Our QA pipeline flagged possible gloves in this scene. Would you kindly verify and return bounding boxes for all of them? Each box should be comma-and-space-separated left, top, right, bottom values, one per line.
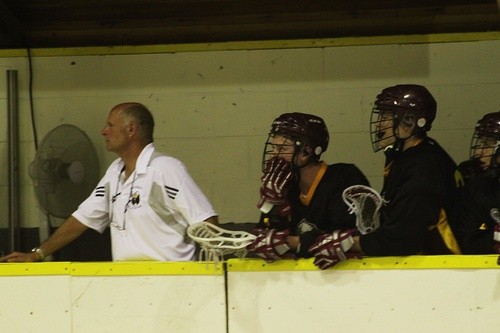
257, 156, 303, 224
246, 228, 298, 264
308, 227, 363, 271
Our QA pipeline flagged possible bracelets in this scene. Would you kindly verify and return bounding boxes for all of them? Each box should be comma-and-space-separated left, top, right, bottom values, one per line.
32, 248, 44, 260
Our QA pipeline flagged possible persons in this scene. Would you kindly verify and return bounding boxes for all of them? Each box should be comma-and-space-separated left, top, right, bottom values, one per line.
311, 84, 477, 270
246, 113, 371, 262
457, 111, 500, 254
0, 103, 219, 262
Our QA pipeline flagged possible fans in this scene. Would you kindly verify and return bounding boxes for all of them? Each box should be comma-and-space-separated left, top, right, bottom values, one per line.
30, 125, 100, 262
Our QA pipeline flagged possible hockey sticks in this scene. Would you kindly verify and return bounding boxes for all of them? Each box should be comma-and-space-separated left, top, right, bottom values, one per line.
341, 184, 385, 235
185, 221, 259, 257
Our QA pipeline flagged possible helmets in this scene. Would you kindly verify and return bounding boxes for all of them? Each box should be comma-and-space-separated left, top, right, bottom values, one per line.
369, 84, 437, 153
262, 112, 329, 173
470, 111, 500, 177
